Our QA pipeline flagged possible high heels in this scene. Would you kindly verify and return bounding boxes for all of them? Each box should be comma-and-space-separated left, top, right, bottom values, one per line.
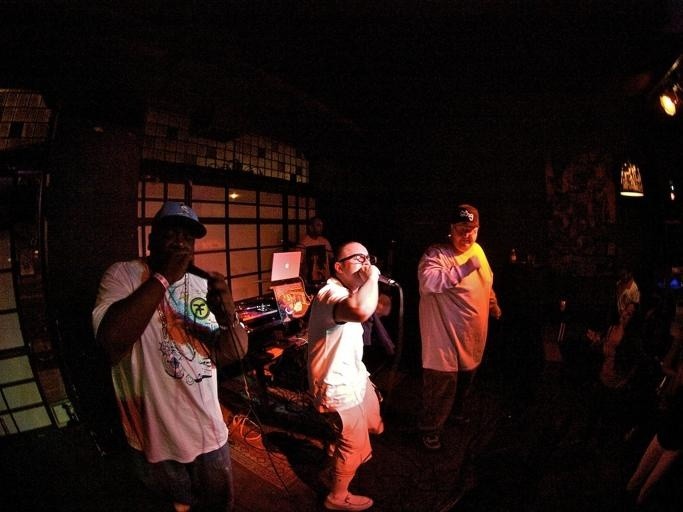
328, 443, 372, 464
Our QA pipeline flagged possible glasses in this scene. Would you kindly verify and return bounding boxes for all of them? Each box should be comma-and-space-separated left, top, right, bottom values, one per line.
339, 254, 377, 265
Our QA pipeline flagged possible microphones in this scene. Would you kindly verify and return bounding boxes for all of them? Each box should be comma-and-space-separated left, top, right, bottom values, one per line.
379, 275, 397, 287
188, 264, 211, 280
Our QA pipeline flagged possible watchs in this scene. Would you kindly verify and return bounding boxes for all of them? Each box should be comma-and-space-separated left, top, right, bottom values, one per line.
219, 312, 240, 331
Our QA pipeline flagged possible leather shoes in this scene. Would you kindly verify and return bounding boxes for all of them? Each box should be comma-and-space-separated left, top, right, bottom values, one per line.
324, 491, 373, 511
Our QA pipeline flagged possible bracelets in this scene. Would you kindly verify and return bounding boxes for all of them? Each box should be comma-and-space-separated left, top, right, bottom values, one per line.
149, 268, 174, 294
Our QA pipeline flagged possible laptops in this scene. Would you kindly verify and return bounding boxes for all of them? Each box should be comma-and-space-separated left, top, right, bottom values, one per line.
252, 252, 301, 282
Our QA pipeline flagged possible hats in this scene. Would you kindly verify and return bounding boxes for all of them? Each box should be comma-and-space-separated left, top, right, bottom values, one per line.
451, 204, 479, 224
151, 202, 206, 239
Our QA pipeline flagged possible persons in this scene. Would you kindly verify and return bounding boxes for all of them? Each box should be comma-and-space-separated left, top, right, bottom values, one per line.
295, 217, 334, 281
306, 240, 392, 512
417, 203, 502, 449
586, 261, 683, 512
85, 199, 249, 512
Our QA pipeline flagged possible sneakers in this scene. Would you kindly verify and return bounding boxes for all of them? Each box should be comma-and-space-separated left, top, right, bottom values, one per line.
453, 415, 470, 422
423, 435, 441, 449
